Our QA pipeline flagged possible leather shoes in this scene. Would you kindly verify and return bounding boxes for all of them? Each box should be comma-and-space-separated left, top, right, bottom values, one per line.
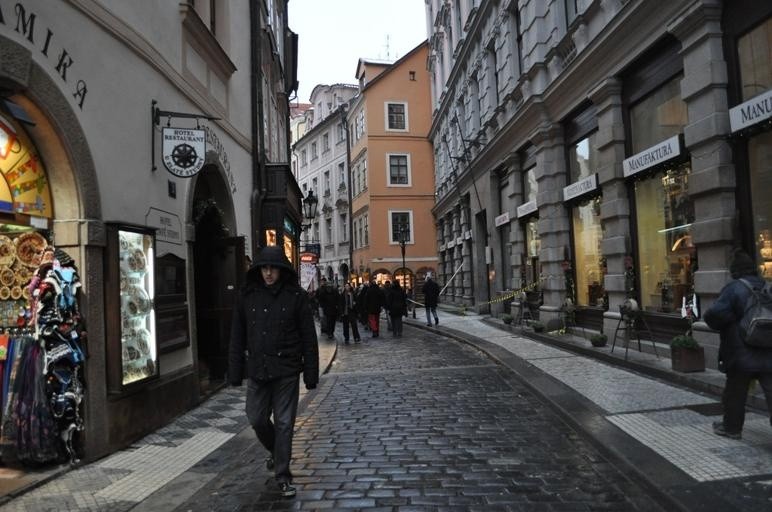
427, 323, 433, 327
327, 333, 334, 339
435, 317, 439, 325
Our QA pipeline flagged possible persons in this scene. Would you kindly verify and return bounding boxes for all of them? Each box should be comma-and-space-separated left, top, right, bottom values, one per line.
422, 277, 440, 327
342, 284, 360, 344
316, 281, 341, 338
702, 255, 772, 438
357, 277, 407, 340
226, 248, 318, 500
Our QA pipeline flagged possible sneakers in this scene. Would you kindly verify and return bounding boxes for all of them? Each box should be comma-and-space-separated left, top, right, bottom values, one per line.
266, 454, 275, 471
712, 421, 741, 440
345, 337, 350, 345
354, 337, 361, 342
278, 481, 297, 497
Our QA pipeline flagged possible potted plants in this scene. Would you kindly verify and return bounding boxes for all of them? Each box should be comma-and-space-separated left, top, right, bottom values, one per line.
591, 334, 608, 346
670, 335, 706, 373
532, 320, 545, 332
502, 315, 513, 324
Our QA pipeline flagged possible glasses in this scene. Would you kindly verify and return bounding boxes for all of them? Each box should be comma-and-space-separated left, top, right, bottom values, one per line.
261, 265, 281, 273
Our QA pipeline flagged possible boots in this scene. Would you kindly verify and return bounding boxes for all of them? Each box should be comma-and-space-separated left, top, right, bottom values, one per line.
372, 331, 378, 338
377, 330, 379, 336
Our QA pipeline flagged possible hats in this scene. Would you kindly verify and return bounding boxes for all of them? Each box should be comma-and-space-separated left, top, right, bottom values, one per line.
326, 284, 332, 286
27, 247, 85, 464
729, 247, 758, 280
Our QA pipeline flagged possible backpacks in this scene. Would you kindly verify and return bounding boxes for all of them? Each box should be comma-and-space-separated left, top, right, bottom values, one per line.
738, 276, 771, 352
